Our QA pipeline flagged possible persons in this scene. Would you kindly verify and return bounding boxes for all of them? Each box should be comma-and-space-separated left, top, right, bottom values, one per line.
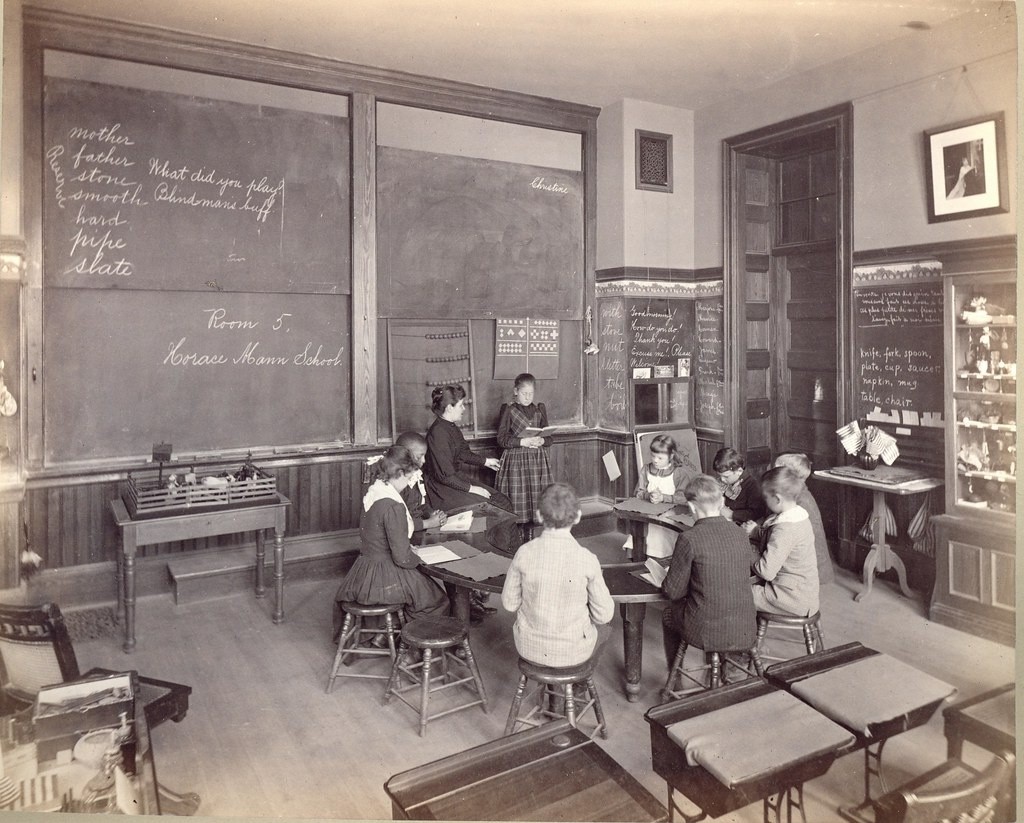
622, 435, 690, 573
660, 475, 757, 700
333, 445, 450, 648
946, 157, 975, 200
495, 373, 554, 538
501, 482, 615, 720
713, 448, 833, 618
397, 431, 498, 626
423, 385, 520, 555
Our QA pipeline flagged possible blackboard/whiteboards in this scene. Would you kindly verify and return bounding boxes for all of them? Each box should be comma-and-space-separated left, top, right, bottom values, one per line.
849, 274, 948, 430
595, 281, 724, 434
15, 0, 603, 475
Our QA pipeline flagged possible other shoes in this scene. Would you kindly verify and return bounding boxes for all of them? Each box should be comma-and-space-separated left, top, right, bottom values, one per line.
371, 634, 389, 649
549, 700, 565, 722
659, 674, 683, 696
402, 649, 422, 683
574, 697, 587, 716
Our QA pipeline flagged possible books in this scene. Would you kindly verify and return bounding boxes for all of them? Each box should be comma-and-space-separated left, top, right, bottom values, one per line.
518, 426, 558, 436
440, 510, 474, 531
641, 558, 670, 588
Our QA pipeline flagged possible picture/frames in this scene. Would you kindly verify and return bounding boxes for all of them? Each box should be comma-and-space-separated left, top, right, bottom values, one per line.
922, 110, 1010, 224
634, 128, 673, 192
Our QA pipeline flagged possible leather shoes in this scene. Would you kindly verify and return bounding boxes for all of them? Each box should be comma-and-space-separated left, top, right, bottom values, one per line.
452, 597, 498, 619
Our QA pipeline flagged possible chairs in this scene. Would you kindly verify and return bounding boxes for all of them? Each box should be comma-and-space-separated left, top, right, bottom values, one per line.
872, 745, 1017, 823
0, 601, 192, 729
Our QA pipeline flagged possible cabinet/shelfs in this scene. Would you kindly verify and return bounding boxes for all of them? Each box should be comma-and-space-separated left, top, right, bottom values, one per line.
930, 269, 1016, 648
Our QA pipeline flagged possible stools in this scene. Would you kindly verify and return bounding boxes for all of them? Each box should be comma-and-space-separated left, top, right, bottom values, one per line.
747, 610, 823, 679
502, 656, 608, 741
660, 637, 763, 704
380, 615, 490, 734
325, 600, 407, 696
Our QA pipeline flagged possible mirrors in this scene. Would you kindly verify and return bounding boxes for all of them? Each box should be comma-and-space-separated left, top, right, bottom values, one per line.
628, 378, 695, 430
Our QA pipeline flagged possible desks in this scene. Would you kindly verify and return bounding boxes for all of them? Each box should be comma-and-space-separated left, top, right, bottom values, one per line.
943, 681, 1016, 759
382, 717, 668, 822
764, 640, 959, 822
644, 675, 857, 822
812, 463, 945, 602
410, 495, 769, 701
109, 491, 292, 653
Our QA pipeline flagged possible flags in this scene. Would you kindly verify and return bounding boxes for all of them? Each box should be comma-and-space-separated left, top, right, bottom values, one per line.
837, 419, 900, 466
